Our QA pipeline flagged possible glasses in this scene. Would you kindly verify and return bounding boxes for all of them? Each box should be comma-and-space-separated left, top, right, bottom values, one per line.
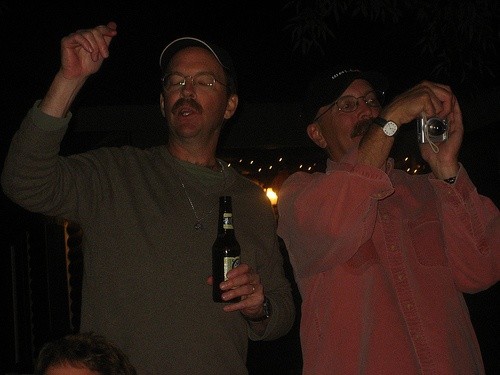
164, 72, 226, 93
313, 91, 386, 124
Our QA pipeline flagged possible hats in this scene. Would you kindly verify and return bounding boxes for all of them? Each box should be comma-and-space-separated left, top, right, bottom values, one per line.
305, 60, 375, 126
159, 36, 237, 89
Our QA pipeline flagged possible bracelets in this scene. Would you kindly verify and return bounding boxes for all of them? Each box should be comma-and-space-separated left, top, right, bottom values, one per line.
444, 176, 457, 184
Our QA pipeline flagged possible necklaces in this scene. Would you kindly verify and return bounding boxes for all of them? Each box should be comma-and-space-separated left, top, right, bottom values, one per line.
175, 175, 219, 232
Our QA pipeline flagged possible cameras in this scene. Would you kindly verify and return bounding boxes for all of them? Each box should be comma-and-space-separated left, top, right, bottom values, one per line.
416, 112, 449, 142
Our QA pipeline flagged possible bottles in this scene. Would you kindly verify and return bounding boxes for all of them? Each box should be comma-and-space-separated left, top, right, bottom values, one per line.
212, 196, 240, 304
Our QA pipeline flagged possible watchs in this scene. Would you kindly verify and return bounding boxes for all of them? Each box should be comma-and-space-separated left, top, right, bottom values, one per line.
372, 117, 399, 137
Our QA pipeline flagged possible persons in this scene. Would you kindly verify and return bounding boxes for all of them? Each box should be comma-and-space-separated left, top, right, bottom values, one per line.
1, 19, 296, 375
33, 328, 137, 375
275, 65, 500, 374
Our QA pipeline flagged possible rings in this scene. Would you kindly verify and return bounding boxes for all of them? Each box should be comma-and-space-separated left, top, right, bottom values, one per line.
251, 285, 256, 293
